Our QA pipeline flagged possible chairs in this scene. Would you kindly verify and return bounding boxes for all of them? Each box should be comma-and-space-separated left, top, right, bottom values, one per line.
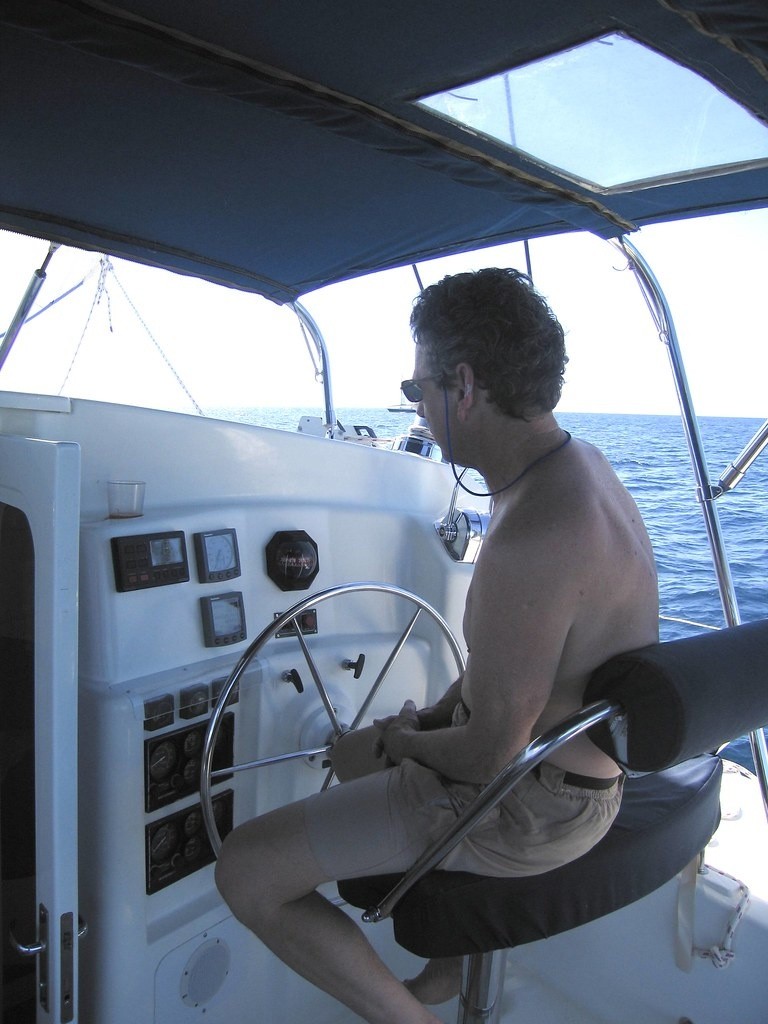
340, 617, 768, 1024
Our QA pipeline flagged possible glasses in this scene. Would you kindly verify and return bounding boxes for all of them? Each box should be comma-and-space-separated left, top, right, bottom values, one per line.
400, 373, 456, 403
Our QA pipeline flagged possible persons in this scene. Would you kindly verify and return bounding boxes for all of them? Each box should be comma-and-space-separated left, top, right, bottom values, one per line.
215, 267, 657, 1024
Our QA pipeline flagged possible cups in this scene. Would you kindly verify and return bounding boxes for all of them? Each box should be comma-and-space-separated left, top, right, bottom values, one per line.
106, 480, 146, 518
